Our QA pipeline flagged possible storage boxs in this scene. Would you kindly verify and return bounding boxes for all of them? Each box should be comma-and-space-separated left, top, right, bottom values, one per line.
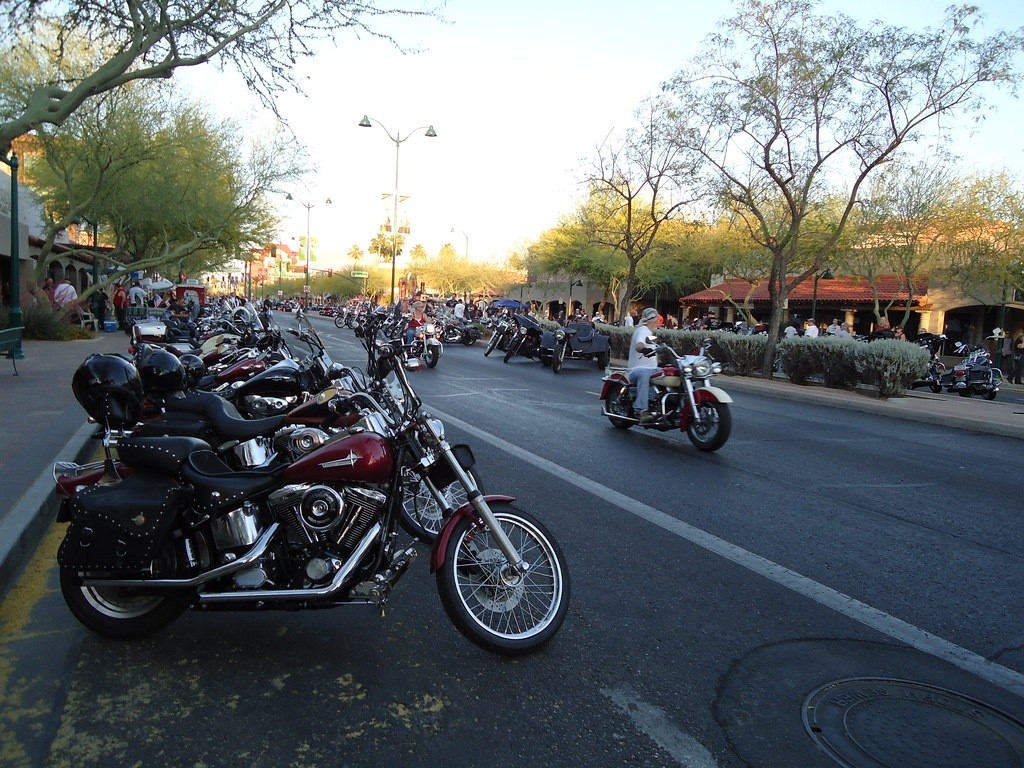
141, 418, 207, 440
58, 482, 188, 574
103, 321, 117, 332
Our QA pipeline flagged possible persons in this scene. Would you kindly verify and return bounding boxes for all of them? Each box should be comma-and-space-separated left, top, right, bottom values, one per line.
111, 283, 128, 330
874, 316, 891, 331
95, 288, 108, 330
825, 319, 852, 339
895, 325, 906, 342
803, 318, 819, 338
454, 299, 465, 326
784, 321, 798, 338
626, 308, 685, 424
485, 302, 496, 317
1006, 333, 1024, 384
263, 294, 273, 313
682, 310, 734, 331
624, 311, 679, 330
129, 281, 147, 306
165, 297, 190, 324
42, 277, 78, 312
551, 308, 605, 327
466, 298, 478, 322
445, 293, 458, 314
998, 329, 1014, 376
394, 291, 435, 345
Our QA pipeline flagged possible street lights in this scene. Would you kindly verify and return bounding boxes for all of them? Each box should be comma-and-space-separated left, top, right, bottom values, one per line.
519, 280, 534, 313
359, 114, 437, 309
449, 229, 471, 273
568, 277, 584, 318
654, 276, 672, 310
278, 234, 295, 290
286, 191, 333, 314
809, 266, 836, 320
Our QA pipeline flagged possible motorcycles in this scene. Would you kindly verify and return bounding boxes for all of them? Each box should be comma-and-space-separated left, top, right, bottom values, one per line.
48, 274, 1005, 657
598, 334, 733, 452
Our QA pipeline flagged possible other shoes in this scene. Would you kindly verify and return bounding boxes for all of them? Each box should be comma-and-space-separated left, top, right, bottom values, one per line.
640, 411, 653, 423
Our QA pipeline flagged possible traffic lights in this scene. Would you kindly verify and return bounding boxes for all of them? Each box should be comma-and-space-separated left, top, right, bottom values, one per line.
327, 269, 333, 278
304, 265, 308, 274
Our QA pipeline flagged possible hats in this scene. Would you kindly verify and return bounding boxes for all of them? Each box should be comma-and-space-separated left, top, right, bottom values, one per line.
65, 277, 73, 283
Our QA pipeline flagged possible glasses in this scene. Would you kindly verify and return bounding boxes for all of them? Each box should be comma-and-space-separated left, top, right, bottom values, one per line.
418, 305, 423, 308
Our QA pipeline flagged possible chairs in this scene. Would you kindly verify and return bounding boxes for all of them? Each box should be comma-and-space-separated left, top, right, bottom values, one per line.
76, 306, 99, 333
0, 327, 25, 376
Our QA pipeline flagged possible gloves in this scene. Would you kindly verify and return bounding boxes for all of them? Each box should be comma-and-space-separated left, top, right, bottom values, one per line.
639, 348, 655, 358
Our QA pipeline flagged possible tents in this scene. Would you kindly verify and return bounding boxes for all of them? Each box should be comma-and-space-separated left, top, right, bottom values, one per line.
486, 299, 530, 309
